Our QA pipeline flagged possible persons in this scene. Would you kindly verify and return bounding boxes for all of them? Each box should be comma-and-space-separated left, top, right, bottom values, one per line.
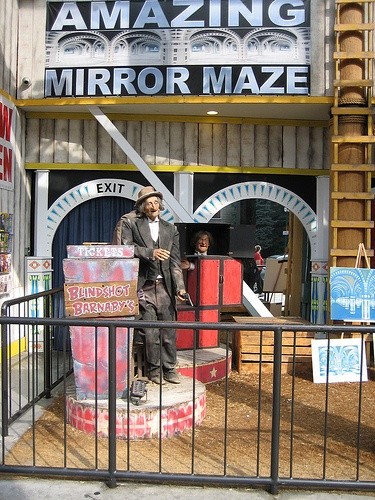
253, 244, 264, 293
180, 230, 222, 256
121, 187, 187, 384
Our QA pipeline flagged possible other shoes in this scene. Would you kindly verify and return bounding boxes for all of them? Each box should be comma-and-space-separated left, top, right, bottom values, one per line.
150, 376, 166, 385
164, 372, 181, 384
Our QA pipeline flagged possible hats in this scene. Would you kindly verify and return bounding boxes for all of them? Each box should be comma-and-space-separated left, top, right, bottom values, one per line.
135, 186, 163, 206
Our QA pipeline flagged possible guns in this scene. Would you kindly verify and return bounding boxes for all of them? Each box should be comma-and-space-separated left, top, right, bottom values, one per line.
181, 293, 193, 306
